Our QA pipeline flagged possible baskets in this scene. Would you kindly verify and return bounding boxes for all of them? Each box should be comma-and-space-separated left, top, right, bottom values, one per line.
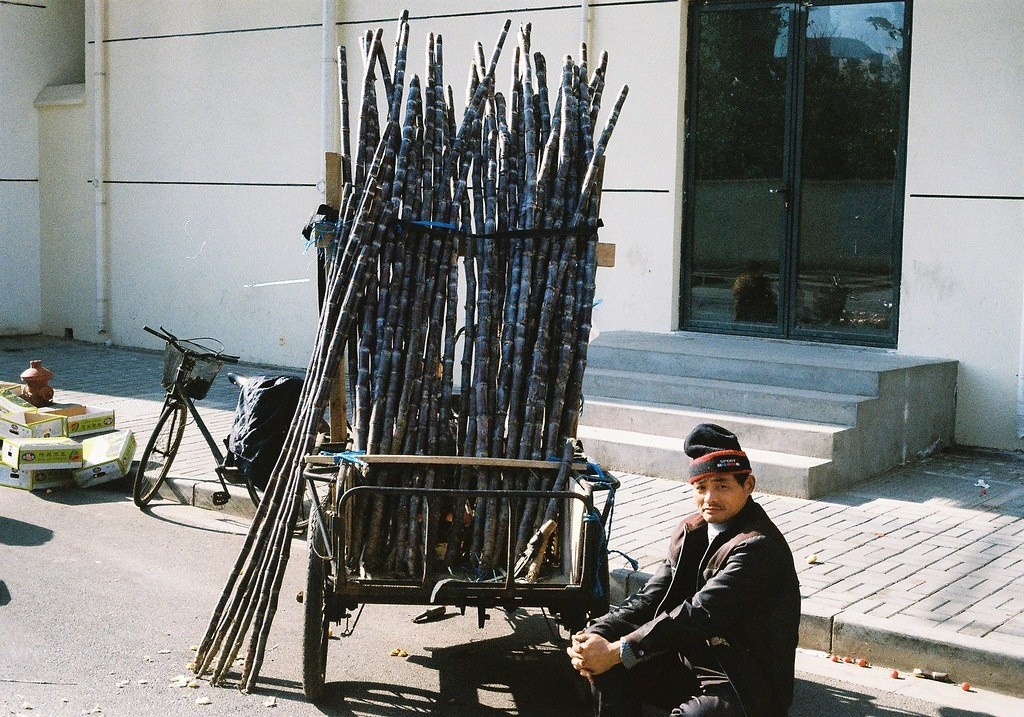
161, 341, 225, 400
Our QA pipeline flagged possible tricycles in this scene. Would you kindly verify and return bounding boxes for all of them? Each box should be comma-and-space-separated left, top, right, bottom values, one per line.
295, 299, 621, 701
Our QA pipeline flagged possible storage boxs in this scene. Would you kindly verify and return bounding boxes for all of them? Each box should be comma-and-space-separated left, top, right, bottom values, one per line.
0, 381, 137, 490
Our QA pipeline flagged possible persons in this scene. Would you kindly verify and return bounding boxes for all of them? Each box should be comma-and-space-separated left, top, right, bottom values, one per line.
565, 422, 801, 717
733, 260, 778, 324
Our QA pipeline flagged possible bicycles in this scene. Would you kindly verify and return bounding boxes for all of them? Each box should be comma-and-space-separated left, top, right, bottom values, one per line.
134, 325, 346, 532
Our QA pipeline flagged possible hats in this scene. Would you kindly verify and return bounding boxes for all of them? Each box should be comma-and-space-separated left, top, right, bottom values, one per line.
684, 423, 752, 485
